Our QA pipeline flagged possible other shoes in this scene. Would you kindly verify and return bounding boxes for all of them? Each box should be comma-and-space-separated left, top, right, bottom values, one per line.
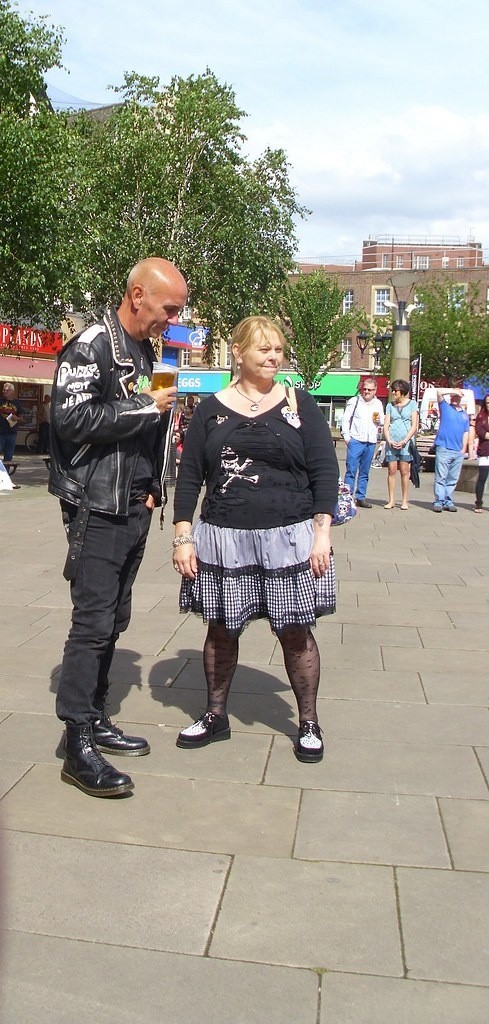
433, 503, 443, 511
384, 501, 395, 509
296, 719, 324, 762
475, 503, 482, 513
357, 499, 372, 508
176, 710, 231, 749
444, 504, 456, 512
400, 500, 408, 510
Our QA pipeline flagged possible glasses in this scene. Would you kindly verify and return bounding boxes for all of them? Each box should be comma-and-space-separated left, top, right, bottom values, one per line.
363, 388, 375, 392
392, 388, 405, 392
2, 389, 13, 392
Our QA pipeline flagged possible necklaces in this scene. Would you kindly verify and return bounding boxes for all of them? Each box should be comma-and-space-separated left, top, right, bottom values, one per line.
235, 381, 273, 411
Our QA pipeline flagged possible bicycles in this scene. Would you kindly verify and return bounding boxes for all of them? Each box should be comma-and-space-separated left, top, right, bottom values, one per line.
25, 430, 40, 452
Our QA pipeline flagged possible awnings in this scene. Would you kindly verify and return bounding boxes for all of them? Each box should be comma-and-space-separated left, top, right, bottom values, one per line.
0, 354, 56, 385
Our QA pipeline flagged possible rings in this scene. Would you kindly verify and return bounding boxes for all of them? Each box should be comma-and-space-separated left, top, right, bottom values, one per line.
319, 561, 323, 565
175, 565, 178, 570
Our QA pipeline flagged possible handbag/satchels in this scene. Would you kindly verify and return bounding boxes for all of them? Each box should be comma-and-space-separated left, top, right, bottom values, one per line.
289, 386, 357, 526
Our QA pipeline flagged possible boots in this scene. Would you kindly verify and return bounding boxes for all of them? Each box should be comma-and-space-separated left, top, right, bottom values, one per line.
96, 694, 150, 756
61, 719, 135, 796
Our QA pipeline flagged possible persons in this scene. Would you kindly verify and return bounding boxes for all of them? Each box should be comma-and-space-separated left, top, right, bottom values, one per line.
0, 382, 24, 472
466, 394, 489, 513
37, 394, 51, 454
48, 257, 188, 798
172, 316, 341, 762
341, 378, 385, 507
432, 388, 470, 512
174, 396, 196, 477
383, 378, 419, 510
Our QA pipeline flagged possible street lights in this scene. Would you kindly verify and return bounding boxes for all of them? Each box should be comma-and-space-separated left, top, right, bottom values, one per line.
355, 328, 393, 379
382, 273, 421, 414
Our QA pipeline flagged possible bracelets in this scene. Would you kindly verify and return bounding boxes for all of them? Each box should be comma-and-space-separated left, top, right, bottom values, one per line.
173, 536, 193, 547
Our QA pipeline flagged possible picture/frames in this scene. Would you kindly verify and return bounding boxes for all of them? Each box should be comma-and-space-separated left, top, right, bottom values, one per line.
16, 400, 39, 431
17, 382, 41, 401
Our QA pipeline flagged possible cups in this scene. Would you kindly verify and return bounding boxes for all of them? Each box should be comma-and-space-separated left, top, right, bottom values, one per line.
373, 412, 379, 422
150, 361, 179, 394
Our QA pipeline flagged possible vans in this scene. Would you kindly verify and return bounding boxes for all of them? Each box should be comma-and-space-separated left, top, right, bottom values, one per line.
418, 386, 476, 433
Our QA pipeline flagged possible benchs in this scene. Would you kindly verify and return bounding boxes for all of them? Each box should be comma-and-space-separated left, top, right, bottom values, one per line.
413, 438, 438, 460
328, 428, 342, 447
